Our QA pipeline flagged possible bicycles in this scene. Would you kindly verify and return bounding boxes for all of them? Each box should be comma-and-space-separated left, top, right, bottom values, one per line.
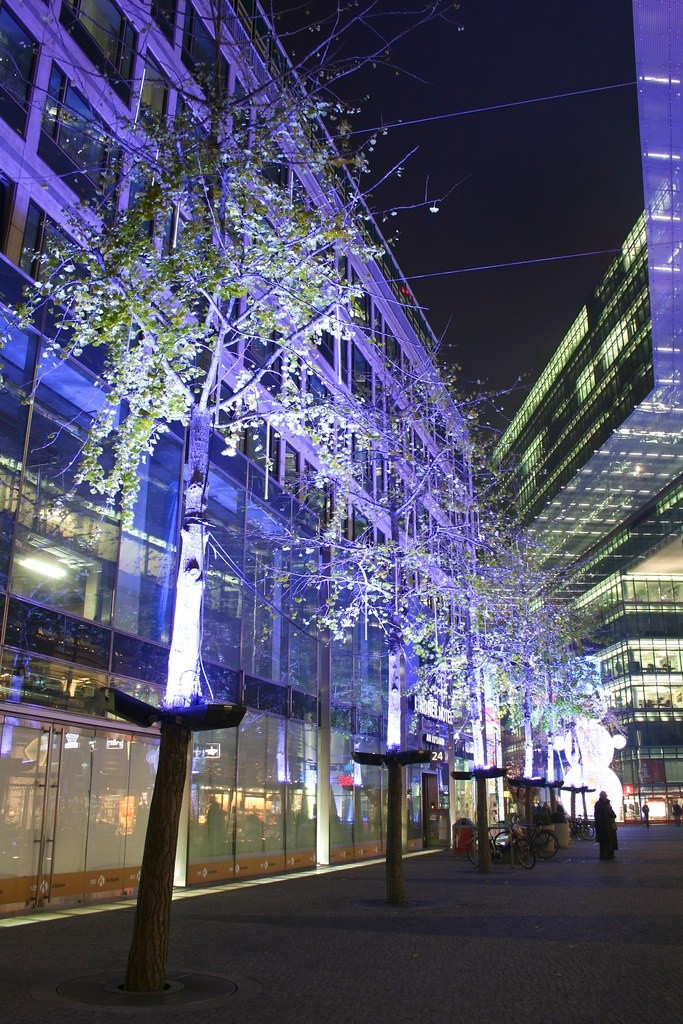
467, 812, 559, 869
568, 815, 596, 841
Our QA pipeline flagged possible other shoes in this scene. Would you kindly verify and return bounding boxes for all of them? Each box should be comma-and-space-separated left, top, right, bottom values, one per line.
599, 855, 616, 860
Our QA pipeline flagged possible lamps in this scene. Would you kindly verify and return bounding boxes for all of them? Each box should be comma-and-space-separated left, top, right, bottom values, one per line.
100, 686, 165, 728
483, 768, 507, 778
351, 751, 386, 765
450, 772, 475, 780
507, 778, 595, 793
394, 750, 433, 765
166, 702, 247, 730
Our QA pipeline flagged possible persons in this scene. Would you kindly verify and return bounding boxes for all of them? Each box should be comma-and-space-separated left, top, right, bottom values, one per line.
537, 803, 542, 815
543, 802, 552, 816
594, 791, 619, 859
531, 804, 536, 816
641, 801, 650, 827
673, 801, 682, 828
555, 800, 566, 818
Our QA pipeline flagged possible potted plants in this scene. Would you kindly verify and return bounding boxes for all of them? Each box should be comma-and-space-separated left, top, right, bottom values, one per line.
533, 811, 555, 853
551, 808, 569, 847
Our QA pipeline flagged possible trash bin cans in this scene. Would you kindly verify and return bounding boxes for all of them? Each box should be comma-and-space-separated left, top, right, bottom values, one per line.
451, 817, 476, 855
